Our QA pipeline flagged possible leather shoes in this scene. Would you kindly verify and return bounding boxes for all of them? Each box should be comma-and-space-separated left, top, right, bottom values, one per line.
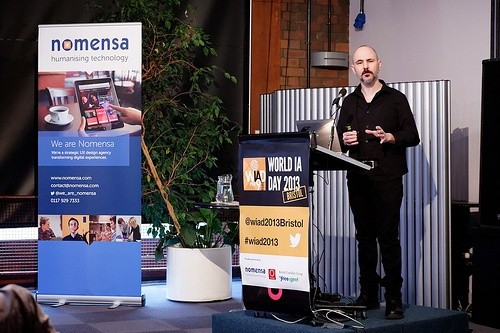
345, 294, 380, 311
385, 299, 404, 320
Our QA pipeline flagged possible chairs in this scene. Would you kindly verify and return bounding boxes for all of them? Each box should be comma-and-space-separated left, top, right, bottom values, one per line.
46, 87, 77, 106
126, 70, 138, 85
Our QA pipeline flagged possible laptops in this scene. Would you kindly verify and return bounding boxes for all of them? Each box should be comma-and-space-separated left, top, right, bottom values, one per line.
296, 119, 346, 155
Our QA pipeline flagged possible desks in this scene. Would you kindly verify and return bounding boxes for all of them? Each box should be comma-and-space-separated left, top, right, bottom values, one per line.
193, 202, 240, 209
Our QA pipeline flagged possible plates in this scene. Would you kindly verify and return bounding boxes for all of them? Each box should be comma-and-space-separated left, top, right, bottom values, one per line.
44, 113, 75, 125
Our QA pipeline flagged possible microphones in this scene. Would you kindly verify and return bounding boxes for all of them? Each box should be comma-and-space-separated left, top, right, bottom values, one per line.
333, 89, 347, 104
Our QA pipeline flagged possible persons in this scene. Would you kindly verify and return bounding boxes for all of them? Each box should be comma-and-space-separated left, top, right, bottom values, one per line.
337, 45, 421, 320
0, 283, 60, 333
41, 215, 141, 242
78, 93, 142, 137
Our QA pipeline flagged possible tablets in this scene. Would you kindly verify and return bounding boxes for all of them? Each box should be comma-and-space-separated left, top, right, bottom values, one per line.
74, 77, 124, 131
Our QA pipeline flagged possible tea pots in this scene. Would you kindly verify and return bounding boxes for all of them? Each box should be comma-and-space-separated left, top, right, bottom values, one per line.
216, 174, 234, 204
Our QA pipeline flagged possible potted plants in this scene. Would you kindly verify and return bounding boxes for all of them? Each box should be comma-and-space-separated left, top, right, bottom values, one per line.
92, 0, 240, 303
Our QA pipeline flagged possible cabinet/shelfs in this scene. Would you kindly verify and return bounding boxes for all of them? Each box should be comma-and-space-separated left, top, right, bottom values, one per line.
38, 71, 67, 103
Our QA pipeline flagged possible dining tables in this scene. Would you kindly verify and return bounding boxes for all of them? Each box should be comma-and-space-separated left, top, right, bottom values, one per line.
38, 103, 142, 137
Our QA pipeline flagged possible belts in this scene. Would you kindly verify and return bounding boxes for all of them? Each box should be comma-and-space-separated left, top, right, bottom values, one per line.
361, 160, 381, 169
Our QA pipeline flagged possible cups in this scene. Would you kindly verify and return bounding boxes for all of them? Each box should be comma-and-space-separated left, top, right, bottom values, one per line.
49, 106, 69, 123
